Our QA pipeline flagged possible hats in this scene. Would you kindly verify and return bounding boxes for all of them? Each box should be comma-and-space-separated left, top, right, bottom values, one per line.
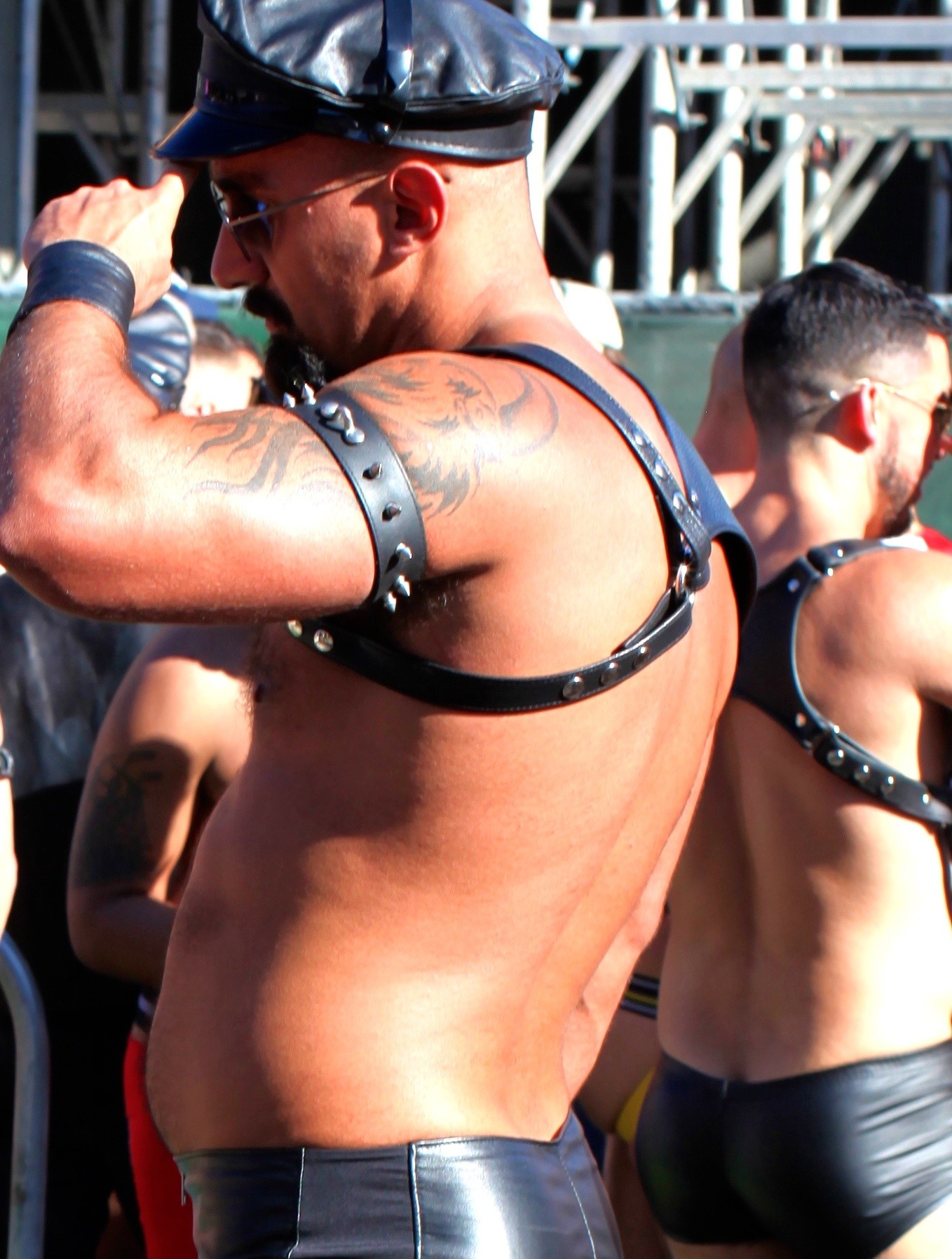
149, 1, 571, 164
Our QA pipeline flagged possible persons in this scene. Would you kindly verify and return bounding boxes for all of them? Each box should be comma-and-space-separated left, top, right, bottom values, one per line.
635, 255, 952, 1259
0, 308, 952, 1259
0, 0, 760, 1259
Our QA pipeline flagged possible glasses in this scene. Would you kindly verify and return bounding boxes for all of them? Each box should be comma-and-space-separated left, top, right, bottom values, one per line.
205, 165, 452, 262
869, 377, 952, 428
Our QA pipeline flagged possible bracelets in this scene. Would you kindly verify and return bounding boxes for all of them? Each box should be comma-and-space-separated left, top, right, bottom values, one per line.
6, 238, 137, 347
